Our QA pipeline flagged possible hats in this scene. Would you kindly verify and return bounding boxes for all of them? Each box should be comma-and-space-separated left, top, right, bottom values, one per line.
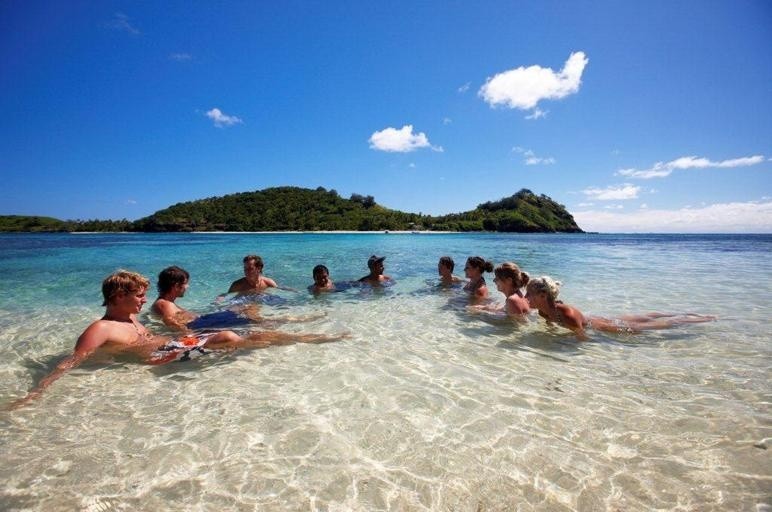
367, 254, 386, 268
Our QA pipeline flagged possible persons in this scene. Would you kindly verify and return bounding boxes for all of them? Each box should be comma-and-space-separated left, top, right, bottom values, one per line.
437, 254, 462, 283
207, 251, 305, 315
524, 276, 723, 343
1, 266, 352, 408
461, 256, 494, 297
464, 262, 532, 321
304, 260, 351, 296
144, 265, 328, 335
357, 255, 396, 288
